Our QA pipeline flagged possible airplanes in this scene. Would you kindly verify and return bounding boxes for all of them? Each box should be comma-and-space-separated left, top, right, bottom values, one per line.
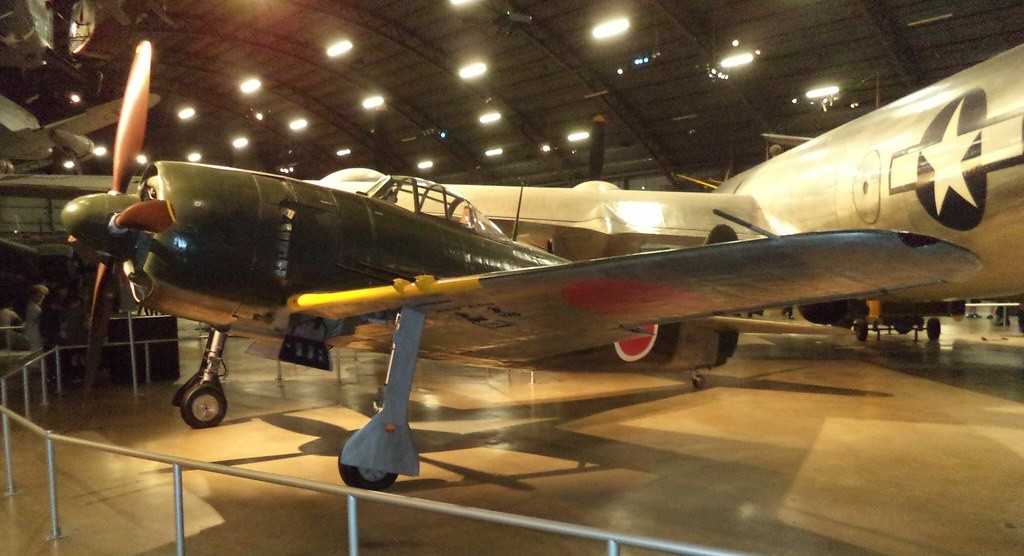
0, 90, 161, 175
58, 36, 988, 491
389, 40, 1024, 343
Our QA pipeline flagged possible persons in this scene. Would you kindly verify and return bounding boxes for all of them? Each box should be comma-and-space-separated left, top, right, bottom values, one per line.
37, 279, 69, 397
781, 305, 794, 319
0, 300, 31, 350
967, 299, 982, 318
986, 306, 995, 319
